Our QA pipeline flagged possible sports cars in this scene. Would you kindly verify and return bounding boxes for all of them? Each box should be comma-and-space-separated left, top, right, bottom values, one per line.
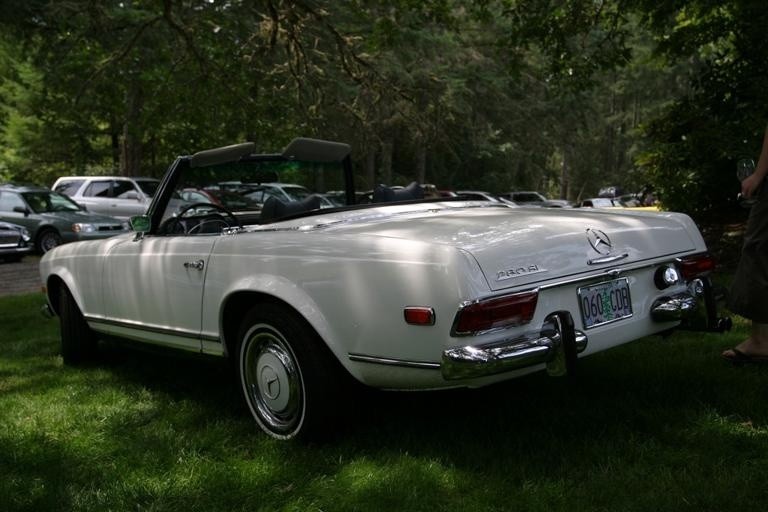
39, 134, 733, 451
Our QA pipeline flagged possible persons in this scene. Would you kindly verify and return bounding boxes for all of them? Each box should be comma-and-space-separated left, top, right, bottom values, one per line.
721, 126, 768, 362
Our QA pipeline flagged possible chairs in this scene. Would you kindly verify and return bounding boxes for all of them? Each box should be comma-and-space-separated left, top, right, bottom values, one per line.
257, 193, 322, 224
372, 180, 425, 205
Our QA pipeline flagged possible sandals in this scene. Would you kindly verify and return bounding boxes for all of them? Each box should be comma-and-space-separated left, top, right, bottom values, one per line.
721, 347, 768, 363
